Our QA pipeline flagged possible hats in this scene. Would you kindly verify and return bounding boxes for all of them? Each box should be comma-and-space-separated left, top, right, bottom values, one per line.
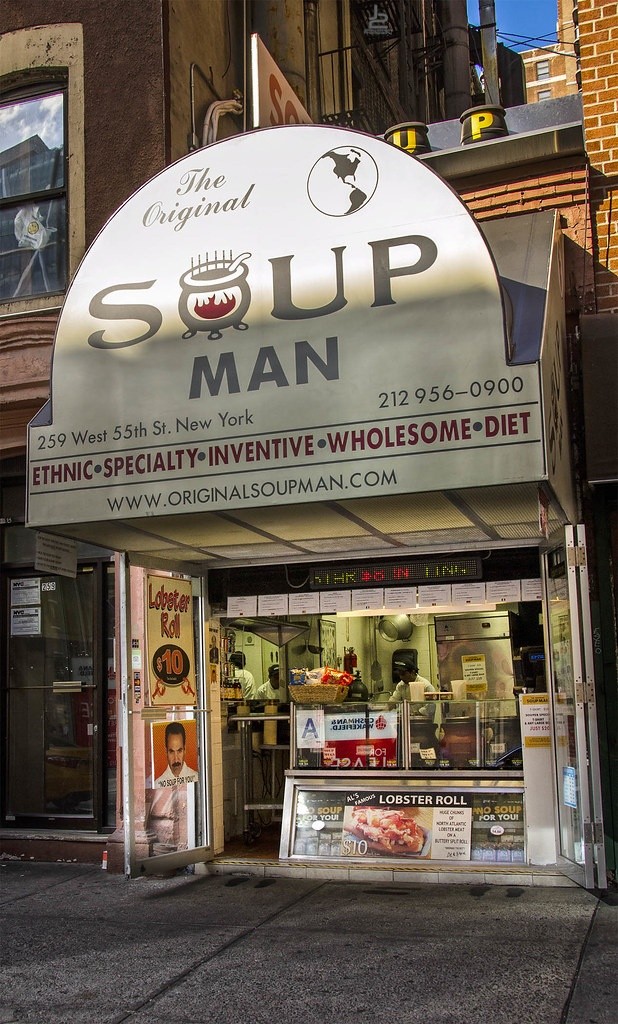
229, 651, 246, 665
268, 664, 279, 677
395, 657, 416, 673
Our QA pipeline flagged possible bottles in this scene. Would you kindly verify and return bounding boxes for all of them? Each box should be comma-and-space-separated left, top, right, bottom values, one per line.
220, 679, 243, 701
346, 678, 368, 702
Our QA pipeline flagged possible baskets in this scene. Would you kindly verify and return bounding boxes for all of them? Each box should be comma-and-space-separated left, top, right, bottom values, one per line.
288, 684, 349, 703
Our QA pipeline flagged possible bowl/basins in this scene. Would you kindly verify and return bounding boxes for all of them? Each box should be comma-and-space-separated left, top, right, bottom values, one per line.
424, 691, 439, 701
439, 692, 453, 700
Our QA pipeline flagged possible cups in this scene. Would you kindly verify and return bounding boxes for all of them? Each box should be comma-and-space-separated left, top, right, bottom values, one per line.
409, 681, 424, 703
450, 680, 467, 701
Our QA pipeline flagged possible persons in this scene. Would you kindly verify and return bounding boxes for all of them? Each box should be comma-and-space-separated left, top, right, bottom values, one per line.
155, 722, 199, 786
256, 664, 280, 702
386, 659, 437, 715
229, 651, 256, 701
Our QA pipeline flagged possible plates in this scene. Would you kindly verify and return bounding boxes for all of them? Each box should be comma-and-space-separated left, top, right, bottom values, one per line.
344, 824, 432, 857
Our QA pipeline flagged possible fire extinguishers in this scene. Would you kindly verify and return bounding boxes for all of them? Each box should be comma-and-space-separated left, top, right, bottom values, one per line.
344, 647, 357, 674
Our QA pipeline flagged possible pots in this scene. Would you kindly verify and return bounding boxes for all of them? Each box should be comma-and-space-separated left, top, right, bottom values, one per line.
379, 614, 413, 642
